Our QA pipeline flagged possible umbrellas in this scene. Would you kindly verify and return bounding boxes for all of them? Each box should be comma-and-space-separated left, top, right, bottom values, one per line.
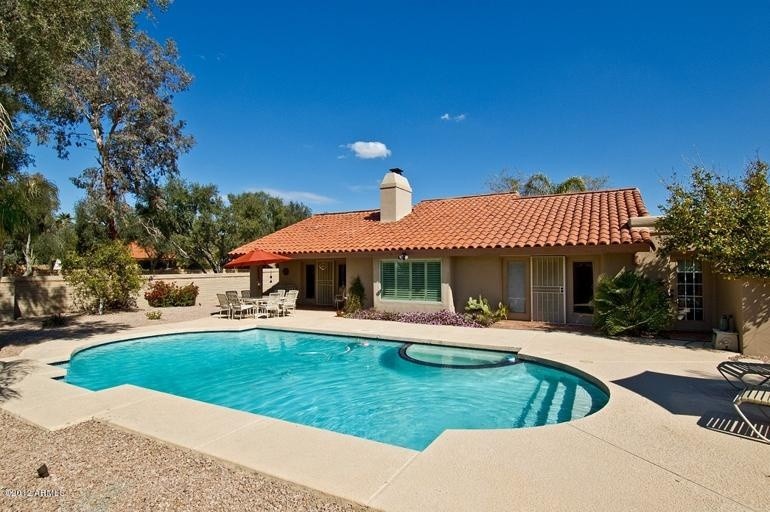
223, 249, 293, 299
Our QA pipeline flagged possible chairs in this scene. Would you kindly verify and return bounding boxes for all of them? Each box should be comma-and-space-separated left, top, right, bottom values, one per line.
732, 388, 770, 444
334, 287, 347, 310
213, 288, 301, 320
716, 359, 770, 390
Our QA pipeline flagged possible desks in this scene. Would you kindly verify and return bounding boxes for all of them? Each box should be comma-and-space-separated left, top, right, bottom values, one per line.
712, 328, 740, 351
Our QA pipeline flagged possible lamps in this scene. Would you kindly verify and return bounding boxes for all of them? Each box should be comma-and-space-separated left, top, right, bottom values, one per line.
398, 250, 409, 260
